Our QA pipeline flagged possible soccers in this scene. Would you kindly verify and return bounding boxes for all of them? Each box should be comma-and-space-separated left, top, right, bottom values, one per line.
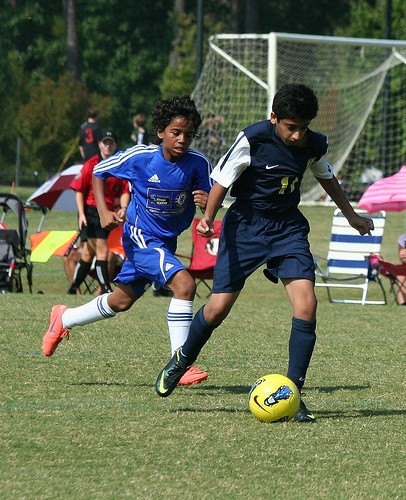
248, 375, 302, 427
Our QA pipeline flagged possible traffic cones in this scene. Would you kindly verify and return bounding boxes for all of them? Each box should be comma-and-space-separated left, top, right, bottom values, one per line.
10, 181, 15, 194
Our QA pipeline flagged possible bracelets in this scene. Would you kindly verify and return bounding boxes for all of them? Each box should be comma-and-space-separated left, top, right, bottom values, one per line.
121, 206, 127, 208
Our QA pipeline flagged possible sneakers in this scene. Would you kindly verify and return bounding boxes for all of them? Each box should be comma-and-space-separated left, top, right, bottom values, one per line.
295, 392, 316, 422
176, 367, 208, 386
156, 346, 193, 397
41, 304, 71, 357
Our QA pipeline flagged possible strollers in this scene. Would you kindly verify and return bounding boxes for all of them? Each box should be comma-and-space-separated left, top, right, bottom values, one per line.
0, 192, 33, 294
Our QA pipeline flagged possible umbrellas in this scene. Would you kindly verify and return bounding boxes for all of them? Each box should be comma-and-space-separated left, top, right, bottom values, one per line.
357, 166, 406, 214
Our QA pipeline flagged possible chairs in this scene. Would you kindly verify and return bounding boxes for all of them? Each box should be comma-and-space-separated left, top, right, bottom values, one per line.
0, 193, 44, 295
186, 217, 223, 299
312, 209, 387, 305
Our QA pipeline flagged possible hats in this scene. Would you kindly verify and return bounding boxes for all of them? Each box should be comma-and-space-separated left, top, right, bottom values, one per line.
97, 129, 115, 142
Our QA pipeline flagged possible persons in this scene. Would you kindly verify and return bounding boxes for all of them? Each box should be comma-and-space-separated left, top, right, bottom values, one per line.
155, 83, 375, 423
41, 95, 225, 386
63, 105, 149, 296
